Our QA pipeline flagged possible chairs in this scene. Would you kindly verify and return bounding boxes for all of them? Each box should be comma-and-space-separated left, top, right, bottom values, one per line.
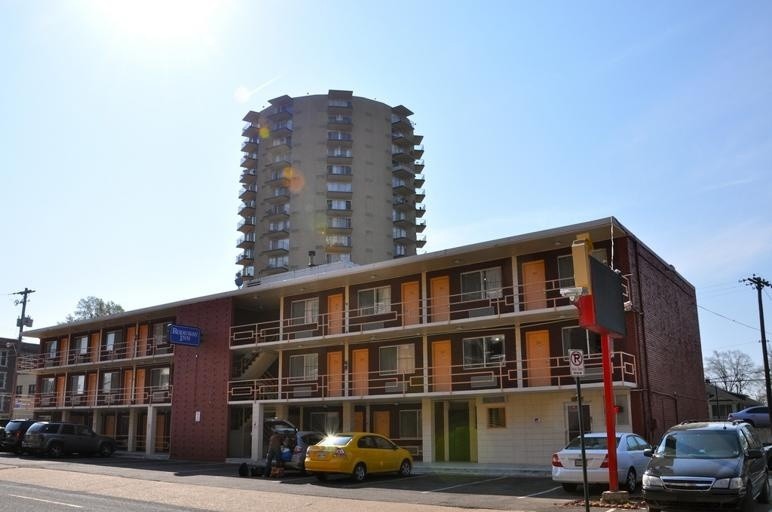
359, 439, 369, 446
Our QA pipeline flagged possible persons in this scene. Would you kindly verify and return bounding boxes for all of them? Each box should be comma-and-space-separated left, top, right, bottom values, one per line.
261, 432, 287, 479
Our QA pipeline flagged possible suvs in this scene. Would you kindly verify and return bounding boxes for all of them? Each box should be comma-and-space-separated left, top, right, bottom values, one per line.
21, 421, 117, 460
0, 419, 50, 457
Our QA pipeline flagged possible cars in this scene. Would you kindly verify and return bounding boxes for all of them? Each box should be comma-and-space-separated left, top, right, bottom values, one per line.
304, 432, 414, 482
728, 405, 772, 428
642, 420, 771, 512
552, 432, 658, 493
263, 417, 329, 477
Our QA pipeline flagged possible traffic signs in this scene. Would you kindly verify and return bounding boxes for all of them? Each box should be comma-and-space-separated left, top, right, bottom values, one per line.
568, 349, 584, 377
